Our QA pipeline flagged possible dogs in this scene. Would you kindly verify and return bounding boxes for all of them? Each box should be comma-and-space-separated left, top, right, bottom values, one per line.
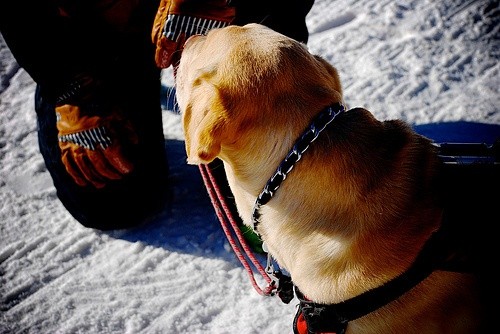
167, 22, 500, 334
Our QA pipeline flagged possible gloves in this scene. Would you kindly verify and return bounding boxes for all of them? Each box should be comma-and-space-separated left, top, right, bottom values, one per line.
152, 0, 236, 77
55, 104, 132, 190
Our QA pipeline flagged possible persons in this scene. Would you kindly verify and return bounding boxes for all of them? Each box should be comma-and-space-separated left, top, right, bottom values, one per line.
0, 0, 315, 231
